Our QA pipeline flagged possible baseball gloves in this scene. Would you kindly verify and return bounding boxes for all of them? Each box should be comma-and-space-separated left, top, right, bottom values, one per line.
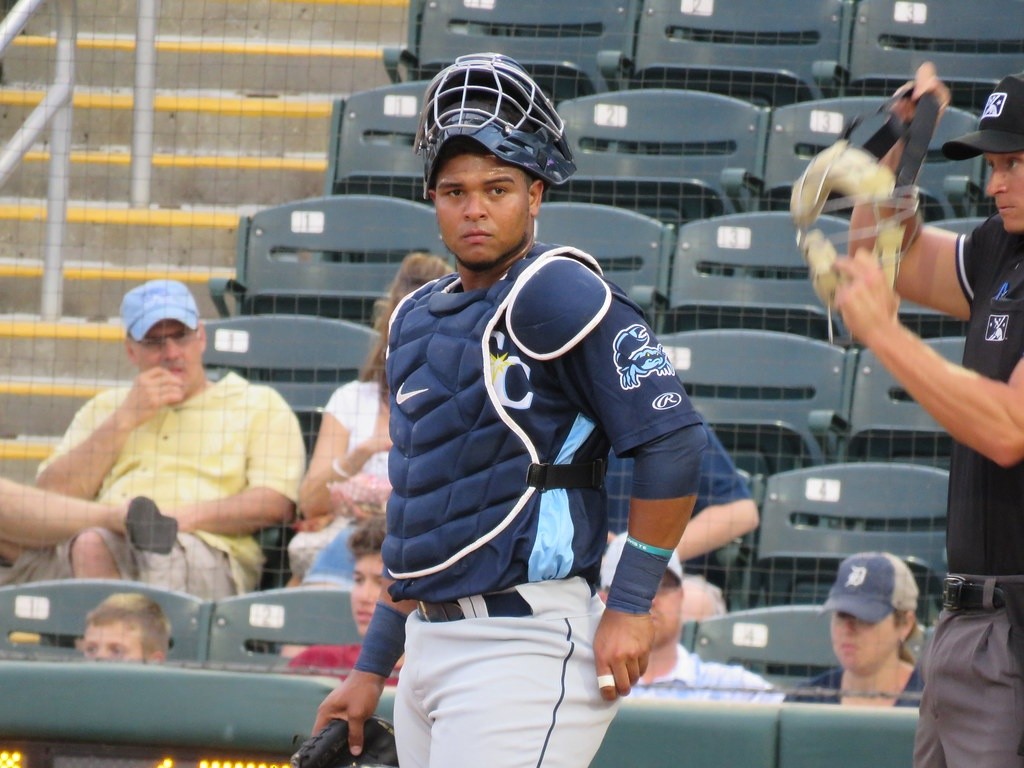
288, 712, 401, 768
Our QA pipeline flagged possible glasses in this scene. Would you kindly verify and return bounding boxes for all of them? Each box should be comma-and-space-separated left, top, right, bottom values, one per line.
139, 329, 198, 347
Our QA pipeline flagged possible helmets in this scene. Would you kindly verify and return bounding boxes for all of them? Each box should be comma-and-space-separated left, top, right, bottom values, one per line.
413, 50, 579, 199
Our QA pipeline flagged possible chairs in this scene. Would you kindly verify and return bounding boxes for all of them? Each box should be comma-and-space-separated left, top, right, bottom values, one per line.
0, 0, 1024, 708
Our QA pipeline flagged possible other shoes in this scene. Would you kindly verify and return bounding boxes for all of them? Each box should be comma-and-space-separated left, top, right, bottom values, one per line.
124, 495, 178, 555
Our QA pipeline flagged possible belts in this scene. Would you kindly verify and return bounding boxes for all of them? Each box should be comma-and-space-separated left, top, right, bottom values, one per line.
416, 575, 598, 622
940, 574, 1005, 613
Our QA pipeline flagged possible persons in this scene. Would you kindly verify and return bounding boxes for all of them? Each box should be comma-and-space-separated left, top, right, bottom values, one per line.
0, 278, 307, 651
285, 515, 415, 689
79, 592, 173, 666
309, 51, 710, 767
599, 530, 785, 708
776, 548, 929, 707
593, 378, 761, 620
281, 249, 460, 656
828, 56, 1024, 768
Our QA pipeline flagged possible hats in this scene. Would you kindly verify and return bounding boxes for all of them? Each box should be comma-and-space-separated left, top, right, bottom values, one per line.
599, 531, 683, 590
816, 550, 920, 622
941, 70, 1024, 161
119, 278, 200, 343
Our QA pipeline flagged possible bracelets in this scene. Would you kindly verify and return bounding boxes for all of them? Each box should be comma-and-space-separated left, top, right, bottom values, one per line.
332, 457, 351, 479
352, 601, 408, 679
605, 534, 674, 615
